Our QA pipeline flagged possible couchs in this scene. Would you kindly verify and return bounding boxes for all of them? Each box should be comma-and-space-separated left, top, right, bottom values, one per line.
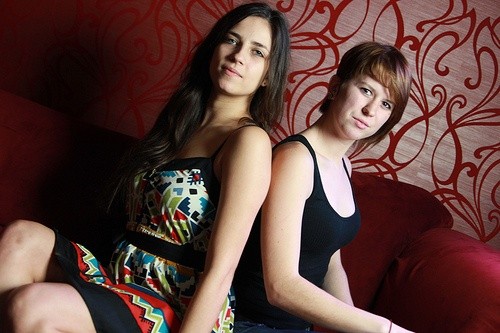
0, 90, 500, 333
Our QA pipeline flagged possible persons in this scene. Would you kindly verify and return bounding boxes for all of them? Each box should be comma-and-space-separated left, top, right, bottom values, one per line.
234, 41, 415, 333
0, 3, 289, 333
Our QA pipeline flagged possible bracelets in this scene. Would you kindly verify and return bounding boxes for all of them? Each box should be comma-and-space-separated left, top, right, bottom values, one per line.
388, 320, 392, 333
